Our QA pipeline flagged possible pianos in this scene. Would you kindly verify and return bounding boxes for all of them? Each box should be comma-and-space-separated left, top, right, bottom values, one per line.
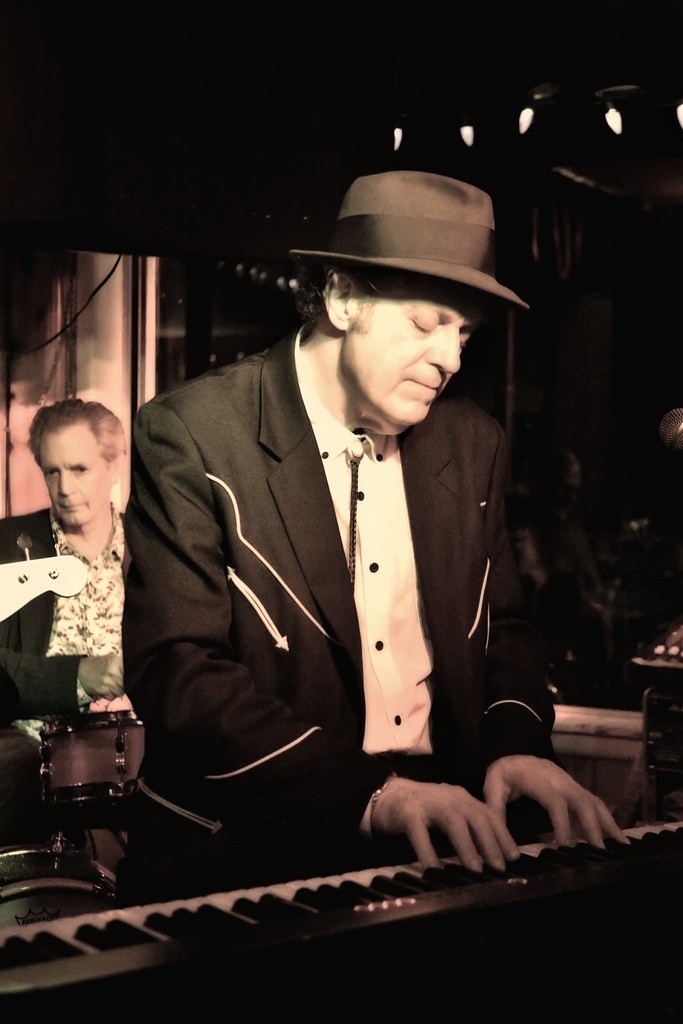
0, 819, 683, 1024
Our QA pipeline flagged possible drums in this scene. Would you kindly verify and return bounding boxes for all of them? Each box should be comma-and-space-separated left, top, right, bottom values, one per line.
1, 842, 119, 928
37, 719, 143, 804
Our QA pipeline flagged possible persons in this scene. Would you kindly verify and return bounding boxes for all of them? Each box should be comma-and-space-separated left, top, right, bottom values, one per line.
124, 170, 630, 915
510, 447, 605, 621
0, 399, 142, 757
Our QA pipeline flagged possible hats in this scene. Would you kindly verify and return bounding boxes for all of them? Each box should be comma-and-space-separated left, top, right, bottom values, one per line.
290, 170, 532, 312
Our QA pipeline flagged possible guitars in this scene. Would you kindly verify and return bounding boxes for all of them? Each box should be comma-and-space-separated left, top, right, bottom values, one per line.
0, 530, 91, 624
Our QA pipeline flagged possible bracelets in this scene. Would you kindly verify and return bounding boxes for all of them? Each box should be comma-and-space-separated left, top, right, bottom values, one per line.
371, 772, 397, 831
